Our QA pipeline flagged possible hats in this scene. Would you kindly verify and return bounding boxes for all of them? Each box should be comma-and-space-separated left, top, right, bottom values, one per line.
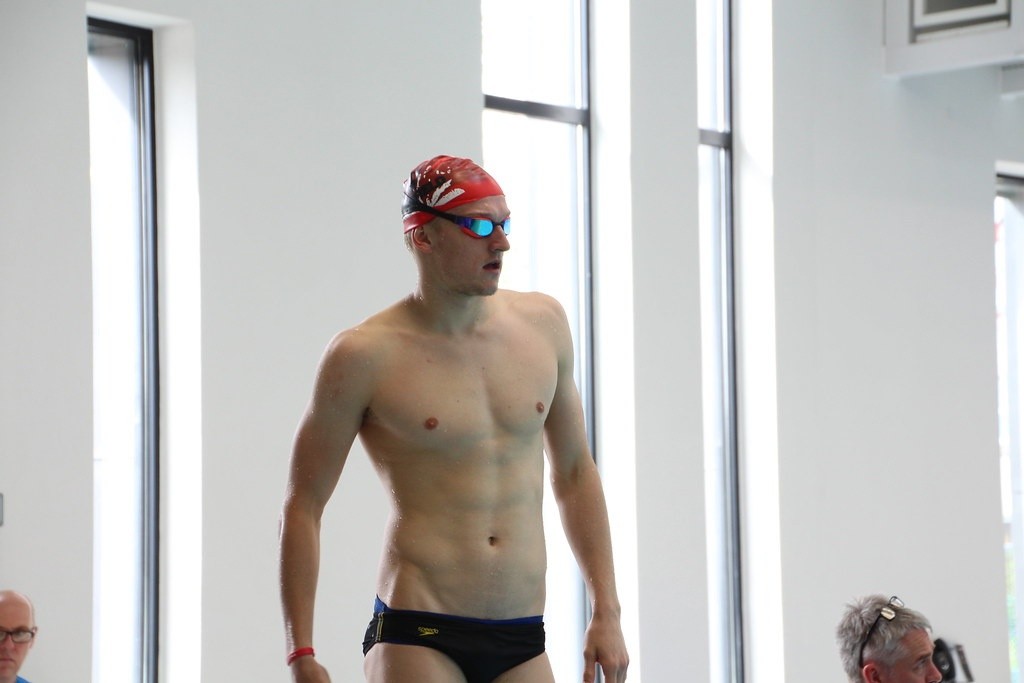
400, 155, 505, 235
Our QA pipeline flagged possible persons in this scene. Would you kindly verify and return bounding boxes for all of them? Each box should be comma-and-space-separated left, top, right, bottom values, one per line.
280, 155, 630, 683
837, 596, 942, 683
0, 590, 38, 683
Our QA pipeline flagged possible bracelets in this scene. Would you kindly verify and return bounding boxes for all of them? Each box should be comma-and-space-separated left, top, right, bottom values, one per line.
287, 648, 316, 666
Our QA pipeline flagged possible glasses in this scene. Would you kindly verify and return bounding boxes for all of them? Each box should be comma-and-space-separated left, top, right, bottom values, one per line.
402, 193, 511, 239
0, 627, 36, 644
857, 595, 905, 669
